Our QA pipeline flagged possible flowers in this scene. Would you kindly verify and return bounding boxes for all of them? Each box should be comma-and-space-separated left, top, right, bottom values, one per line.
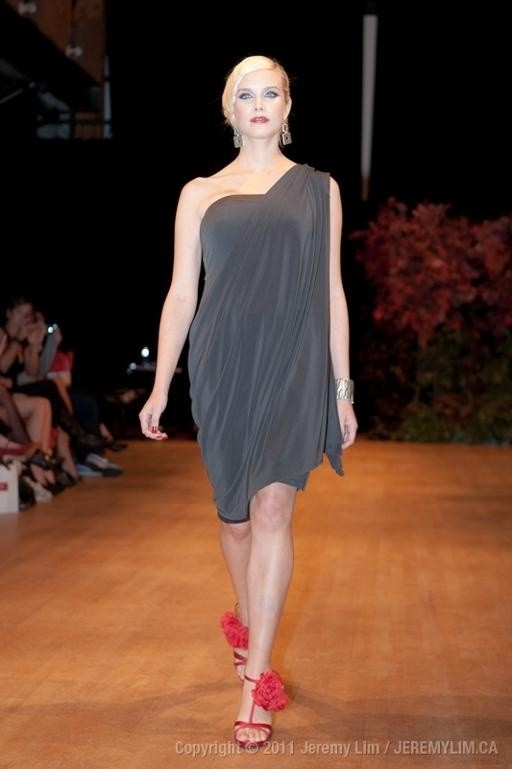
216, 610, 251, 649
253, 669, 288, 711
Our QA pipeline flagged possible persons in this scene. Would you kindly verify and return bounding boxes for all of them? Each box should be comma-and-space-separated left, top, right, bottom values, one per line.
0, 299, 130, 514
134, 50, 363, 752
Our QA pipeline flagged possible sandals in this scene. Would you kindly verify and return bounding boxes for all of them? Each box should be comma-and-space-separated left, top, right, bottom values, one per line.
229, 666, 291, 750
220, 603, 250, 678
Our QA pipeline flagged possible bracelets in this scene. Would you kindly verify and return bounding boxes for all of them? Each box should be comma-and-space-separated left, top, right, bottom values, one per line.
333, 375, 356, 405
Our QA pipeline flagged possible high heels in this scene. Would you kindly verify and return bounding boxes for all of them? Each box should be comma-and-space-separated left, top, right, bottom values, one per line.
21, 430, 131, 510
